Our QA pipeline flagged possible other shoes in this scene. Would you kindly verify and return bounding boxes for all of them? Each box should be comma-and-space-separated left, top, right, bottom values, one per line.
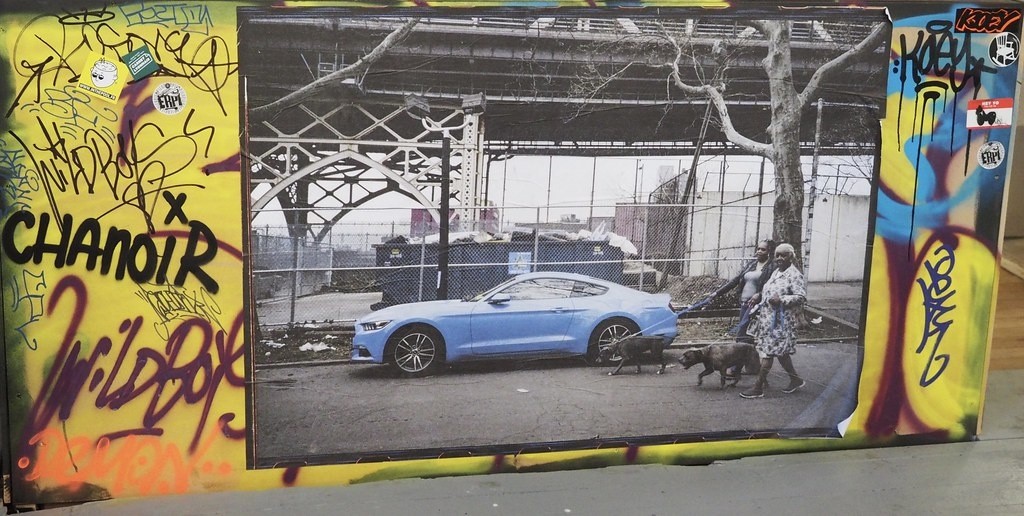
742, 369, 759, 374
781, 378, 806, 393
740, 389, 764, 398
725, 372, 740, 379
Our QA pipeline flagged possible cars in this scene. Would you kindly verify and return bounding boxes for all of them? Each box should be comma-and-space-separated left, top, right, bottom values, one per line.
350, 271, 679, 378
251, 265, 285, 299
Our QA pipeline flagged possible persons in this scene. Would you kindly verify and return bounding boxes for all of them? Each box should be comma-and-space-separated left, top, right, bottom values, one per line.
709, 240, 778, 374
739, 243, 805, 397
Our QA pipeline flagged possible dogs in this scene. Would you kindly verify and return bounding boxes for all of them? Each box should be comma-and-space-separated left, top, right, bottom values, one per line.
678, 342, 769, 390
595, 335, 668, 377
